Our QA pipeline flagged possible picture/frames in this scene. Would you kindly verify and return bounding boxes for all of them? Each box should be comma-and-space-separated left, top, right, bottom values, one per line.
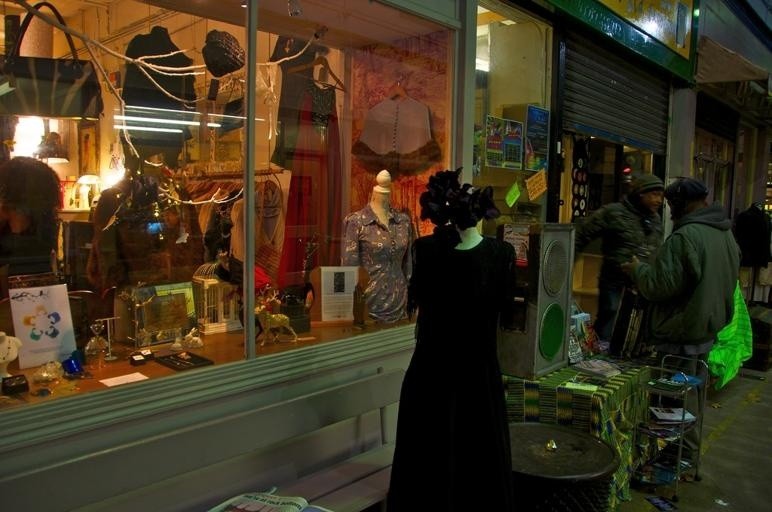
134, 281, 199, 348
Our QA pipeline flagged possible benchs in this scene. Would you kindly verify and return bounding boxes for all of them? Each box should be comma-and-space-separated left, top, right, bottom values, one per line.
69, 402, 400, 511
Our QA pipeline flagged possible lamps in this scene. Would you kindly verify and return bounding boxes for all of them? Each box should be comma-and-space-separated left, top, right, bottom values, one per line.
76, 175, 99, 208
33, 120, 69, 163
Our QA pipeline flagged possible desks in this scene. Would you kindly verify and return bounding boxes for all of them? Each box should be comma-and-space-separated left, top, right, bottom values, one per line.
509, 422, 620, 512
57, 208, 91, 275
501, 354, 651, 512
0, 319, 415, 409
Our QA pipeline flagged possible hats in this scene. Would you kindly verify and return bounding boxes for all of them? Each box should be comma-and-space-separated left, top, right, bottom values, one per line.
202, 30, 245, 78
661, 177, 709, 198
629, 173, 664, 197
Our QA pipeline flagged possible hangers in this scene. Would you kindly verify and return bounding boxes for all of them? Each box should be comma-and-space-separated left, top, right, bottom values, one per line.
387, 81, 408, 99
288, 42, 345, 92
184, 169, 278, 202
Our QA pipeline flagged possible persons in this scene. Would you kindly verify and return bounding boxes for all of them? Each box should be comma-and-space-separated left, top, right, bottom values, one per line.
33, 131, 70, 167
2, 158, 64, 282
570, 172, 668, 347
368, 166, 519, 511
340, 167, 416, 324
93, 128, 209, 316
617, 177, 743, 484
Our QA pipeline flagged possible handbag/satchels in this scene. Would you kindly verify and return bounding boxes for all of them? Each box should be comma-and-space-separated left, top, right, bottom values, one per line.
222, 79, 244, 134
0, 2, 104, 120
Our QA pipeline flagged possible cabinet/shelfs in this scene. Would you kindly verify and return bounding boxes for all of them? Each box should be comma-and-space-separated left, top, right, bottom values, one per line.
631, 354, 709, 503
